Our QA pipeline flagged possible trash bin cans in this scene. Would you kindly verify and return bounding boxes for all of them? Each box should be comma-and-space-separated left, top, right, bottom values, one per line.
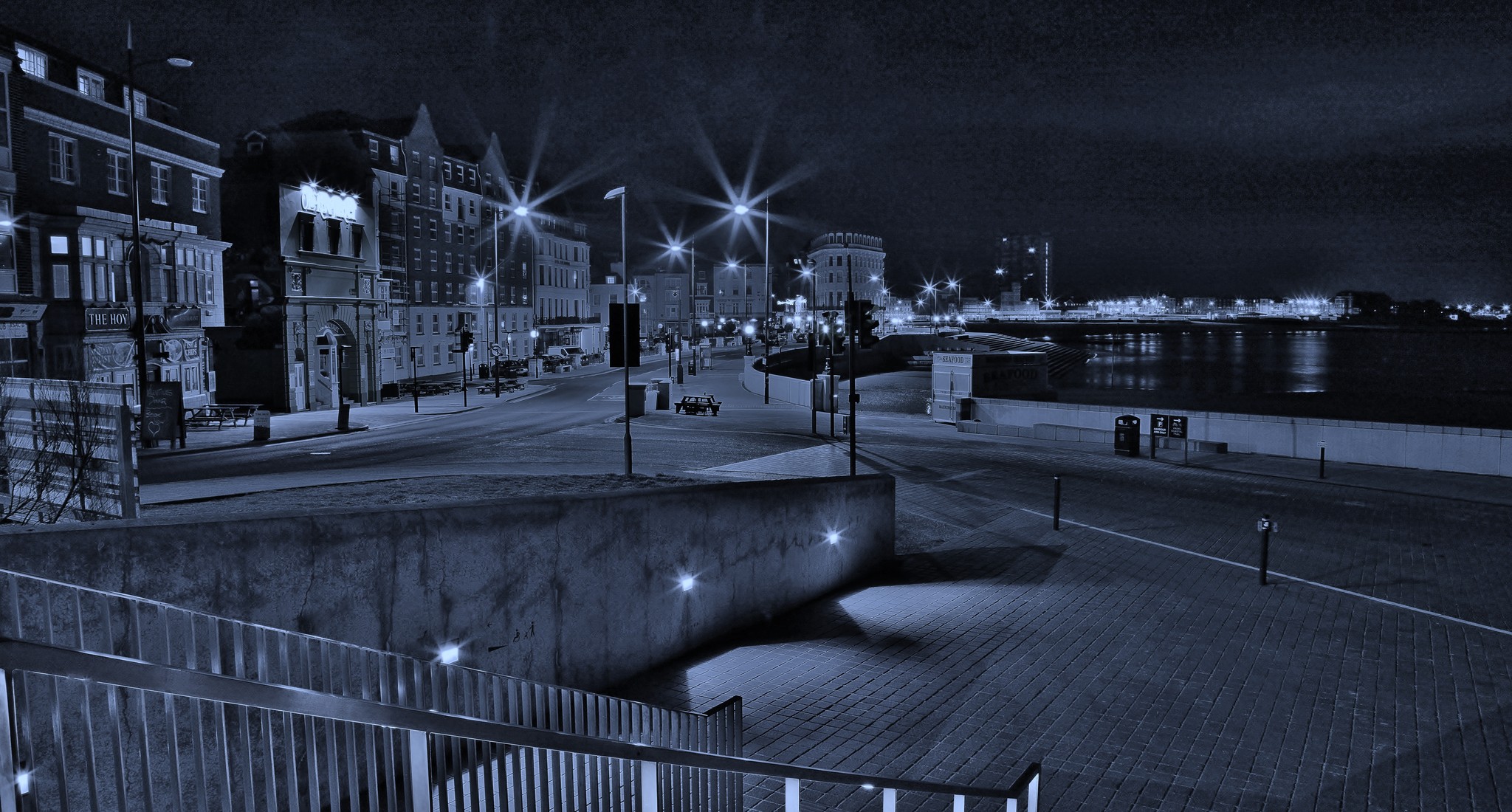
1113, 414, 1141, 458
478, 363, 489, 379
688, 359, 694, 375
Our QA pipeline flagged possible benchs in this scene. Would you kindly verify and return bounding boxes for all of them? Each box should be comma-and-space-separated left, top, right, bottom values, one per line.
186, 413, 254, 430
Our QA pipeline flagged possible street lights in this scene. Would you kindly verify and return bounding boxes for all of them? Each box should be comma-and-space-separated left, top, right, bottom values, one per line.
670, 232, 699, 353
111, 51, 195, 452
928, 285, 933, 334
732, 200, 771, 408
531, 329, 539, 357
871, 275, 885, 337
791, 254, 821, 377
602, 321, 609, 349
601, 180, 636, 475
672, 285, 684, 384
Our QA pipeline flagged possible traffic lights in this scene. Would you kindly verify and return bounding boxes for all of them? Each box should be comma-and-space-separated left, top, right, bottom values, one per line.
831, 323, 846, 357
464, 330, 474, 347
856, 297, 880, 351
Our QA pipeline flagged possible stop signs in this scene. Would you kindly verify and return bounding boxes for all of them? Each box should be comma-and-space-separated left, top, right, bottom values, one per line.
506, 333, 512, 344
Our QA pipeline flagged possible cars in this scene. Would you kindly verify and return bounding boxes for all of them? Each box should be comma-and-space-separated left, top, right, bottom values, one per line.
490, 359, 529, 378
548, 353, 572, 368
536, 354, 560, 372
514, 356, 548, 374
765, 335, 788, 346
796, 333, 809, 343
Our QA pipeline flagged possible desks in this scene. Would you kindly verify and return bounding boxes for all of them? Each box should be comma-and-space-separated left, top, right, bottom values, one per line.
674, 392, 724, 417
206, 403, 264, 428
185, 406, 240, 429
476, 378, 526, 394
399, 380, 461, 398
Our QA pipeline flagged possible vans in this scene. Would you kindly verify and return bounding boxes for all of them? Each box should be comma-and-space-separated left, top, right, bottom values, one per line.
548, 345, 588, 363
